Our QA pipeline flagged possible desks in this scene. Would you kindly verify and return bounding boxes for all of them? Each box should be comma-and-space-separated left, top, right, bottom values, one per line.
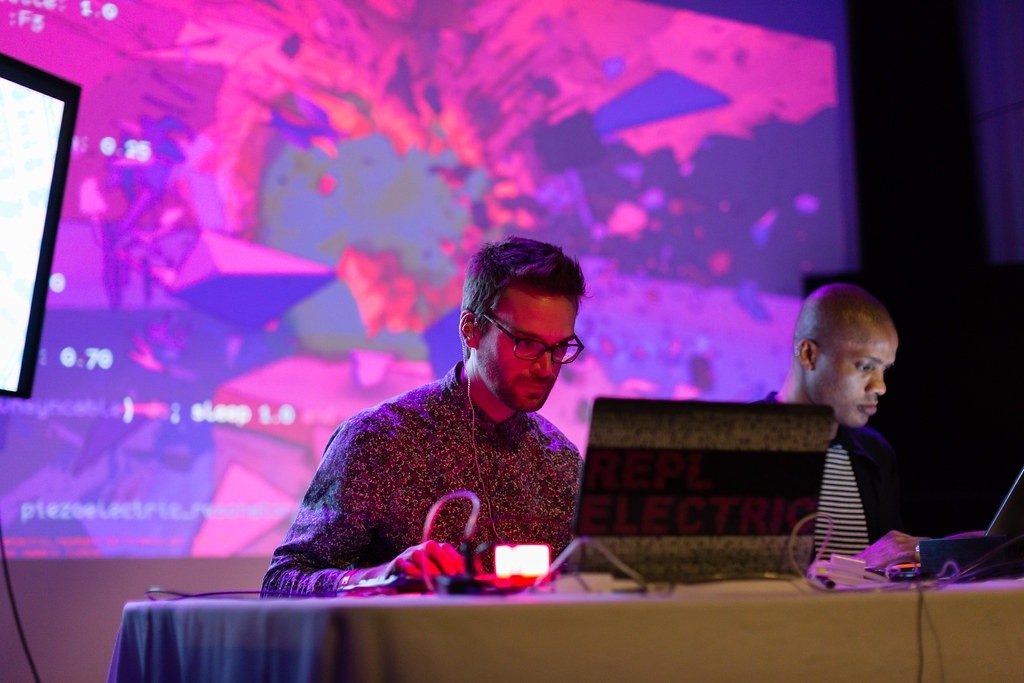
105, 579, 1024, 683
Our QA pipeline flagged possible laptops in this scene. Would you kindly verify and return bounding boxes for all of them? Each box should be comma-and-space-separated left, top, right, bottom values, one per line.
874, 467, 1024, 576
556, 398, 835, 578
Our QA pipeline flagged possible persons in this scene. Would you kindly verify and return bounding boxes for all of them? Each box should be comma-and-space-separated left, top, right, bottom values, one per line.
754, 283, 930, 568
259, 234, 584, 601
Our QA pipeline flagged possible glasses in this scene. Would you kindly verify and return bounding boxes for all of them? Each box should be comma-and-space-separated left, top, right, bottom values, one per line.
481, 311, 585, 364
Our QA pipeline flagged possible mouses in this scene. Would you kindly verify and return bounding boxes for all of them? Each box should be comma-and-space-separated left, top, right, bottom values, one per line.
389, 569, 429, 594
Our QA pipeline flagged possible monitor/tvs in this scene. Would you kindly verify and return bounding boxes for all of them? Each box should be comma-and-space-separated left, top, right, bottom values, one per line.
1, 51, 85, 399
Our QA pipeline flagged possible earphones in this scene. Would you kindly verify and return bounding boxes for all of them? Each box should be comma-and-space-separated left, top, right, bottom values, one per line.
465, 328, 474, 340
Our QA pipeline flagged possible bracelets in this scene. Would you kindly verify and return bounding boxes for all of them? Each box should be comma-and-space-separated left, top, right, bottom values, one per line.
341, 568, 358, 586
